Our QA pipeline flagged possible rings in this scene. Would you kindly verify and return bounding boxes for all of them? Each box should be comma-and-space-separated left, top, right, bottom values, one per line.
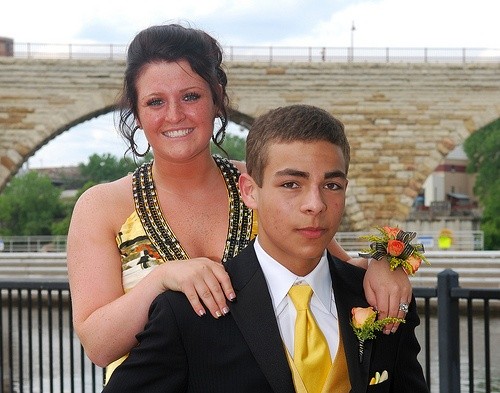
400, 302, 410, 314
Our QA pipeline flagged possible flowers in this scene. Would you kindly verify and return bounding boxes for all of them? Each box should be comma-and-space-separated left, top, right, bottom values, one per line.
349, 307, 405, 363
358, 225, 430, 276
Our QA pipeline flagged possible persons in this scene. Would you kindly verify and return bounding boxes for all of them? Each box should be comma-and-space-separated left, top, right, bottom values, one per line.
100, 104, 434, 393
65, 24, 411, 387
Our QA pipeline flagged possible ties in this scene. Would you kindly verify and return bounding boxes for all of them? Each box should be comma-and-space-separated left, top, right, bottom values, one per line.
288, 284, 332, 393
